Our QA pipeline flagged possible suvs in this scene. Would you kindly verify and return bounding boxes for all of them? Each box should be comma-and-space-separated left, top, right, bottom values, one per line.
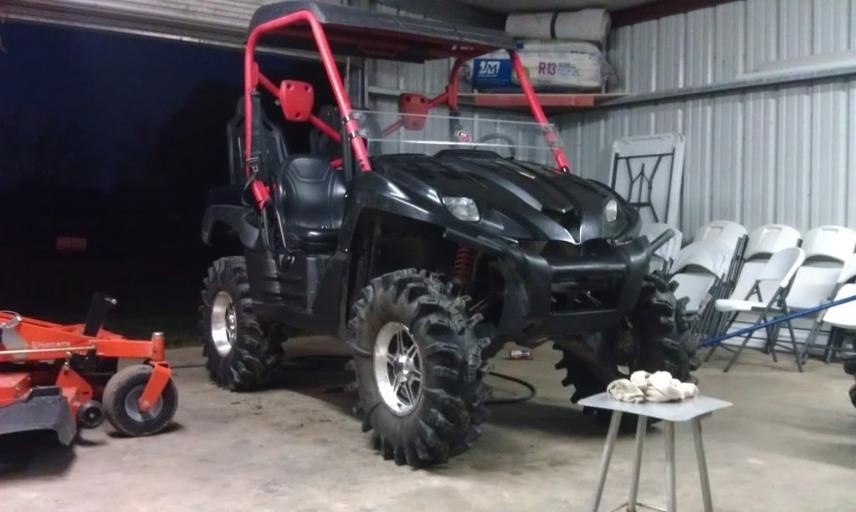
196, 0, 703, 470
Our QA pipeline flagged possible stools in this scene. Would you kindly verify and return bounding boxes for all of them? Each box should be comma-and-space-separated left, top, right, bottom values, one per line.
579, 384, 734, 512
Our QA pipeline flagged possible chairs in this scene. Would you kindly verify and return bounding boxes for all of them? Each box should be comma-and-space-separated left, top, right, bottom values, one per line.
284, 157, 357, 252
631, 217, 856, 374
